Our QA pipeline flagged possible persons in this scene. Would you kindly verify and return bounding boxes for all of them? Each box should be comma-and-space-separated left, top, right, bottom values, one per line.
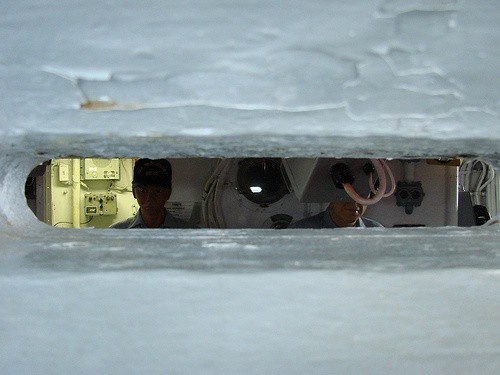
285, 201, 386, 229
105, 159, 196, 228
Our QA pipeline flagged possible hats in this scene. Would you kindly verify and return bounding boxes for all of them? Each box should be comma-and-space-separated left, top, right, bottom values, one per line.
132, 158, 173, 187
